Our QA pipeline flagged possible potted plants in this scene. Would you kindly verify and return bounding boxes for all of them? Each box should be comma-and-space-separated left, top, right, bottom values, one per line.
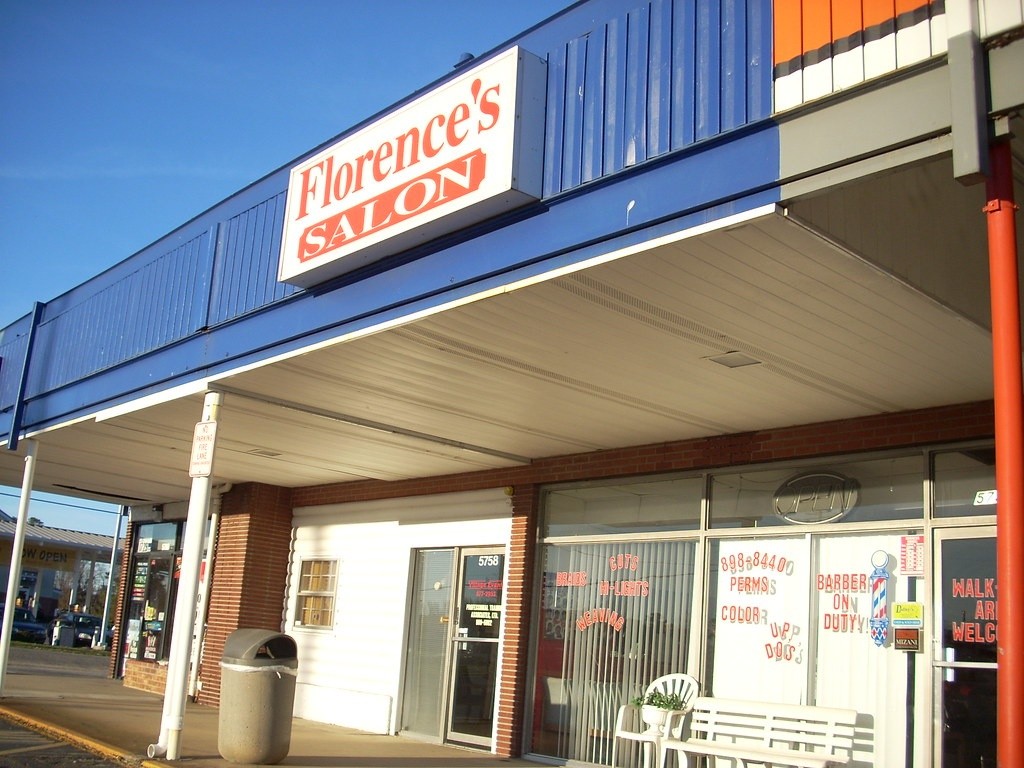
630, 692, 685, 736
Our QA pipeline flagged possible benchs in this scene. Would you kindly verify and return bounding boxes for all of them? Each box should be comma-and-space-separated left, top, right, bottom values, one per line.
660, 697, 858, 768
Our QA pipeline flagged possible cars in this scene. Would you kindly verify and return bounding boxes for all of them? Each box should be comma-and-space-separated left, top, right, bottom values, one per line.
46, 613, 113, 646
0, 603, 47, 644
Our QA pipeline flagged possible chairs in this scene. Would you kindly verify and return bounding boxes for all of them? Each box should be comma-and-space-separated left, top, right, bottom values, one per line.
612, 673, 699, 768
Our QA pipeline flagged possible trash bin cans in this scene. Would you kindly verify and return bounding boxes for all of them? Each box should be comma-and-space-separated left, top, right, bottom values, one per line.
217, 627, 298, 764
52, 618, 75, 647
